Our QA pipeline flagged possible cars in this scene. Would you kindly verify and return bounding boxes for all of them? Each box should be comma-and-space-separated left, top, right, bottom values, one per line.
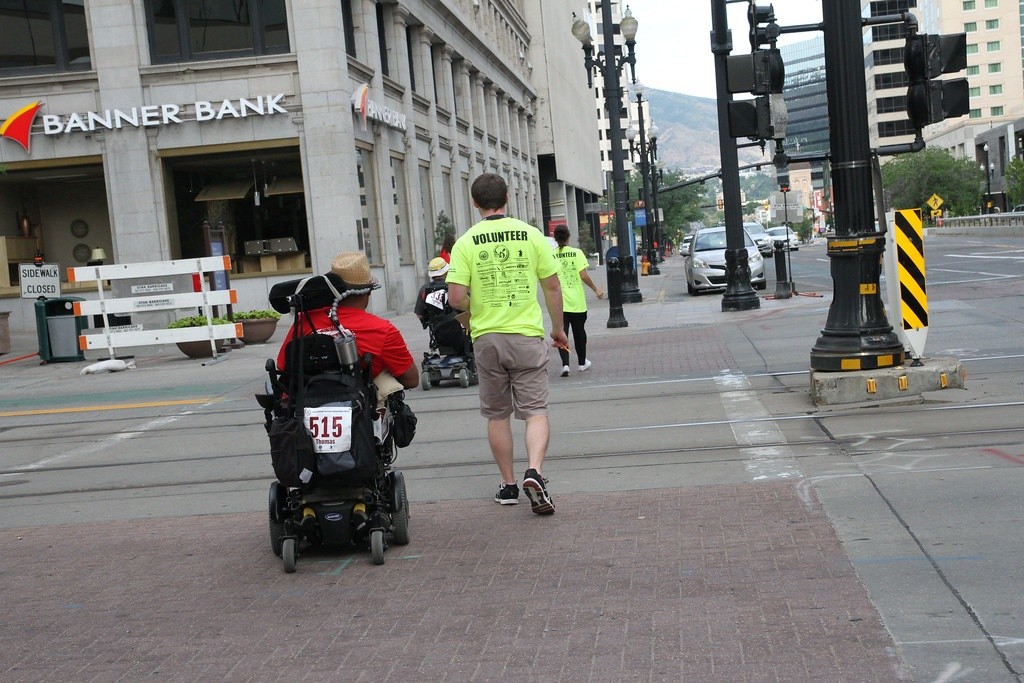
681, 226, 766, 295
766, 226, 799, 251
742, 222, 773, 258
1012, 204, 1024, 214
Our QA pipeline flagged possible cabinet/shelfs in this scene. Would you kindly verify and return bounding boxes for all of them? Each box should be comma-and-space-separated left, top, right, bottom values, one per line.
0, 235, 38, 288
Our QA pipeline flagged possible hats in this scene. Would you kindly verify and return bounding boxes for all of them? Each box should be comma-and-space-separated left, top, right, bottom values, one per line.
428, 257, 449, 277
332, 251, 378, 287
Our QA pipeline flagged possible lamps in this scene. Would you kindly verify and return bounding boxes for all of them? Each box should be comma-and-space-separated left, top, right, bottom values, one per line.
251, 158, 269, 206
91, 247, 106, 266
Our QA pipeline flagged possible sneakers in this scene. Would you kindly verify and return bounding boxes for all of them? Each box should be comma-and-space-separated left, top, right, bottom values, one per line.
523, 468, 555, 515
495, 482, 519, 504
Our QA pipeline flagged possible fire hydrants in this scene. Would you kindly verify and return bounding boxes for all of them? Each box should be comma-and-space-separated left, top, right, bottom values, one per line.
641, 253, 651, 275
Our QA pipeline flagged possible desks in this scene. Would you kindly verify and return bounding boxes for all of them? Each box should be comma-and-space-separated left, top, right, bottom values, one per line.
239, 250, 307, 274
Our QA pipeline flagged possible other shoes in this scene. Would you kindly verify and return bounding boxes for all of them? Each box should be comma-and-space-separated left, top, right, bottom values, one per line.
579, 359, 591, 371
560, 366, 569, 377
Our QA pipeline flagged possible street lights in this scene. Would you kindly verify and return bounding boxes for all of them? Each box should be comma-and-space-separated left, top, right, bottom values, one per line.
571, 0, 642, 304
980, 143, 995, 214
625, 93, 660, 275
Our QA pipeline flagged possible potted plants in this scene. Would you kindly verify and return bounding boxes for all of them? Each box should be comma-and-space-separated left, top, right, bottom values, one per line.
168, 316, 233, 358
222, 310, 282, 345
578, 220, 597, 270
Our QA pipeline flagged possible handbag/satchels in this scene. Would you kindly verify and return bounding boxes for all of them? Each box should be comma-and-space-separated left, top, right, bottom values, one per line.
389, 390, 417, 449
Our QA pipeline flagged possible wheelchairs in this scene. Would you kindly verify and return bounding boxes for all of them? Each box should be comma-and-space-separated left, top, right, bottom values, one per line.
421, 286, 479, 390
255, 271, 410, 574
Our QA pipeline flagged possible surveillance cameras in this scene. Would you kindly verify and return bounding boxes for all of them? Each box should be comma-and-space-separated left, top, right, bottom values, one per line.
354, 85, 368, 112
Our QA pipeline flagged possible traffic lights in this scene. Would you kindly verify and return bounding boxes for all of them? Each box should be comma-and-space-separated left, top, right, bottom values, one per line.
747, 3, 777, 52
903, 32, 971, 129
726, 49, 788, 140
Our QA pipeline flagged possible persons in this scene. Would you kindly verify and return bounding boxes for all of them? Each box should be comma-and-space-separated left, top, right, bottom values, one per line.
445, 173, 568, 516
276, 251, 420, 389
552, 225, 604, 378
414, 256, 474, 354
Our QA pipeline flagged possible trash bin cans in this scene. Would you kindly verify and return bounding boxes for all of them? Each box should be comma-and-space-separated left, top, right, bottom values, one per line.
34, 298, 88, 364
